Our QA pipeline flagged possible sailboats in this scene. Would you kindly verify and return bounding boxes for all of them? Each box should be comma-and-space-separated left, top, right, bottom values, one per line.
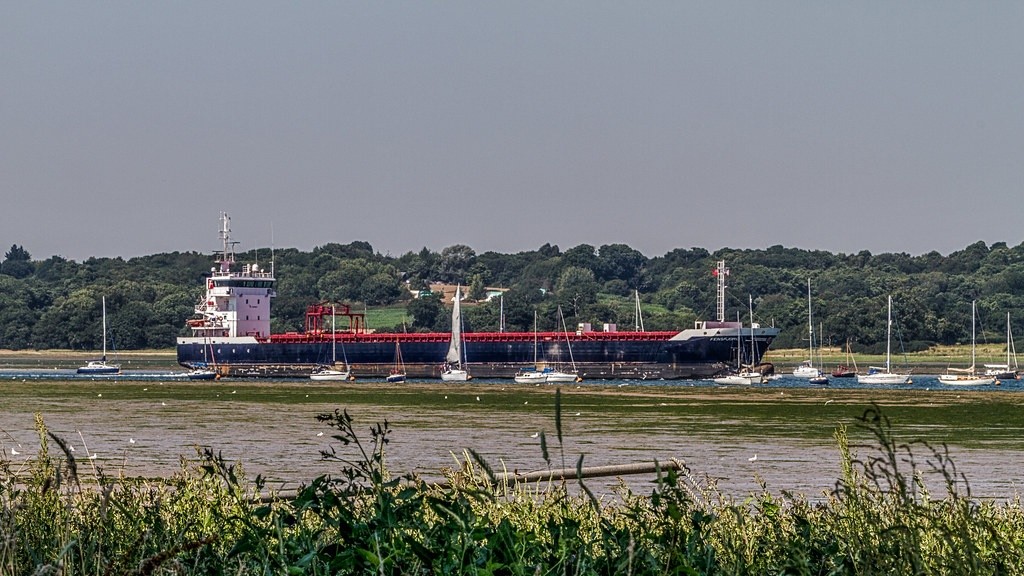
548, 305, 582, 386
440, 282, 471, 382
309, 306, 351, 381
77, 295, 123, 374
514, 311, 548, 384
711, 275, 856, 385
388, 333, 408, 383
857, 295, 913, 385
186, 315, 218, 380
987, 310, 1021, 379
938, 298, 999, 386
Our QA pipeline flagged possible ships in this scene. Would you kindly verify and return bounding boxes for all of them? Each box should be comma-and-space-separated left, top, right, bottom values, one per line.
171, 209, 782, 384
